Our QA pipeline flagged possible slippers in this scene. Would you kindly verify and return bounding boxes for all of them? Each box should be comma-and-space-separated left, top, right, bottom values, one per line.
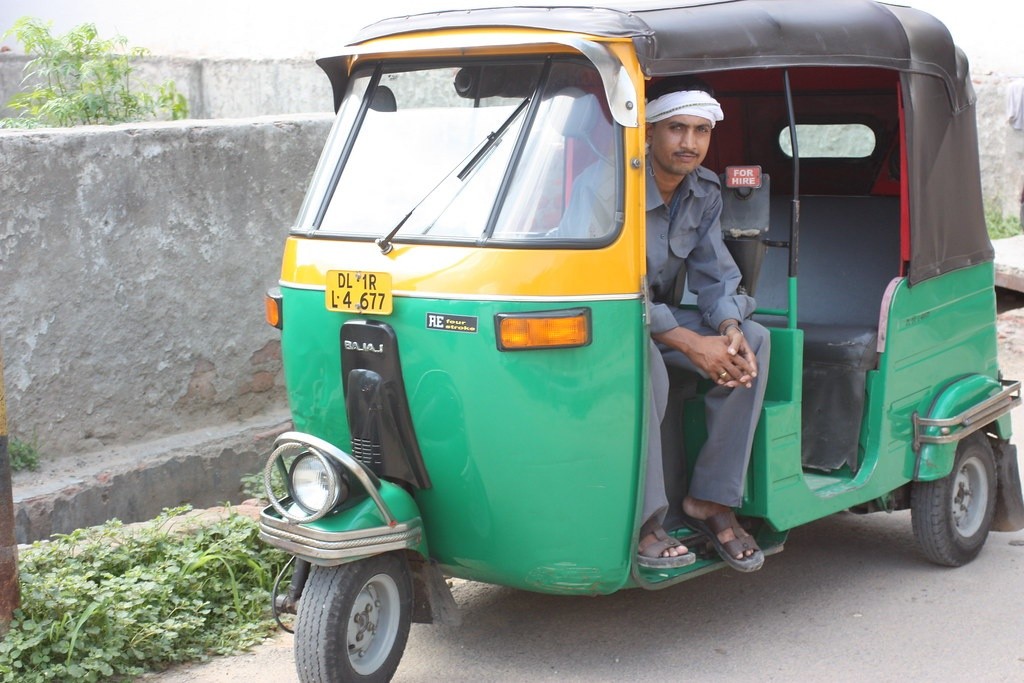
681, 506, 764, 574
633, 520, 699, 569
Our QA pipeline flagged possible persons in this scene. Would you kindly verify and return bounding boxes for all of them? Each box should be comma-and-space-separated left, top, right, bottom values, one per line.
541, 75, 766, 576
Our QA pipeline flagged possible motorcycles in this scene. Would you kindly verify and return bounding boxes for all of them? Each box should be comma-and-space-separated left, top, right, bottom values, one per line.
248, 0, 1017, 681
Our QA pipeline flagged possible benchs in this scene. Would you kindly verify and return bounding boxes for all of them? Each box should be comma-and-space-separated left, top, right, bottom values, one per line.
750, 194, 903, 474
